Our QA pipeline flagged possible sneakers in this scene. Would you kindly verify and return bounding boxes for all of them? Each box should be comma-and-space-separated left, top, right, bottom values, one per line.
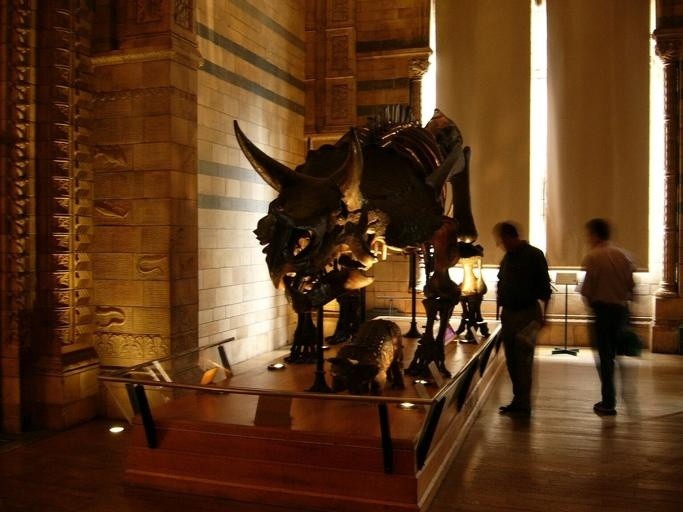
593, 402, 617, 415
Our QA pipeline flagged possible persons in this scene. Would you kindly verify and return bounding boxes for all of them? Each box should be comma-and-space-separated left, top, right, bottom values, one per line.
491, 221, 552, 414
579, 217, 642, 414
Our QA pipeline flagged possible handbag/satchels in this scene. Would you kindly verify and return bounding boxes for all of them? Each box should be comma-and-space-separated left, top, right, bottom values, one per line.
617, 331, 641, 356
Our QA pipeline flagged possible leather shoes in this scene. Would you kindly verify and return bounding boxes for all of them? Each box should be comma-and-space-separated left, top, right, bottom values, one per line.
499, 404, 530, 412
